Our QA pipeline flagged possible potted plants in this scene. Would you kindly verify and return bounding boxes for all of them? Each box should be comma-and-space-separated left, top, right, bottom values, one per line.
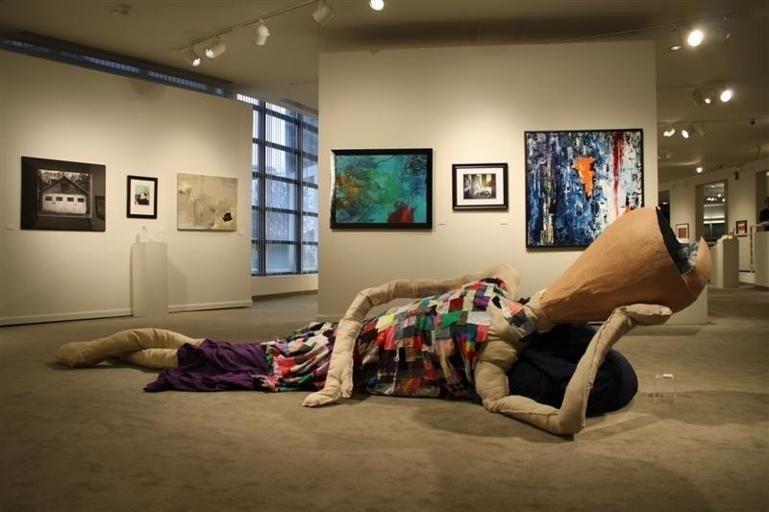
21, 155, 106, 232
127, 175, 157, 218
330, 150, 431, 229
452, 163, 508, 210
176, 173, 237, 231
521, 128, 645, 248
735, 220, 747, 237
675, 223, 689, 243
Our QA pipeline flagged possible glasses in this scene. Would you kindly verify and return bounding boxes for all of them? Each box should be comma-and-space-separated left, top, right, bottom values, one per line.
368, 0, 384, 11
700, 124, 709, 136
312, 0, 333, 24
182, 46, 202, 67
670, 32, 683, 51
205, 35, 226, 59
666, 127, 676, 138
253, 18, 271, 46
704, 95, 716, 105
710, 24, 731, 44
689, 90, 702, 107
681, 124, 697, 138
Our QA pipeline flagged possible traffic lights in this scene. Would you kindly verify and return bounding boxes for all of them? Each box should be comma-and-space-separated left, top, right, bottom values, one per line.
511, 321, 637, 417
